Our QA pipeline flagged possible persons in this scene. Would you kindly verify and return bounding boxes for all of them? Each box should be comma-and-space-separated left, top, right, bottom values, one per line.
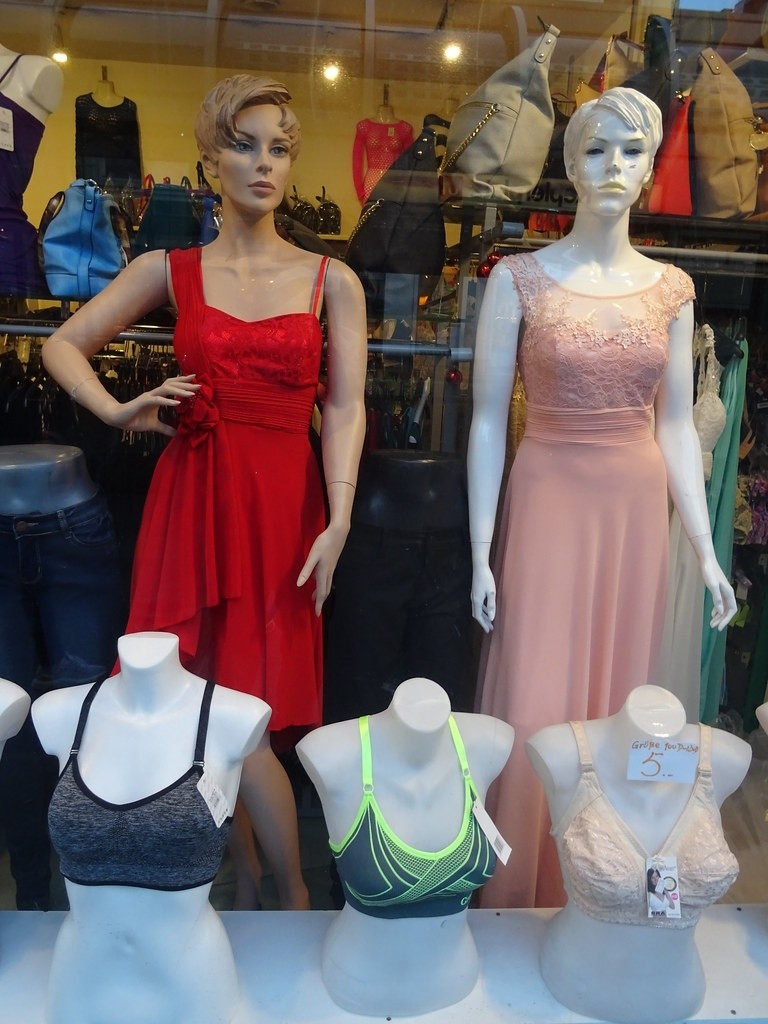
352, 106, 414, 207
524, 684, 751, 1024
0, 41, 64, 299
296, 677, 516, 1018
43, 73, 368, 910
647, 868, 676, 917
422, 98, 460, 195
30, 633, 271, 1024
468, 86, 739, 909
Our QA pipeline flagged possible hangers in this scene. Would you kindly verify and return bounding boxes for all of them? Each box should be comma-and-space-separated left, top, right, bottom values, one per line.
0, 333, 172, 418
694, 299, 749, 363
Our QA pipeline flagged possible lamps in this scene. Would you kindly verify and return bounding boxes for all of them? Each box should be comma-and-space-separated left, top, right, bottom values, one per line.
48, 14, 69, 64
444, 4, 461, 60
323, 31, 339, 80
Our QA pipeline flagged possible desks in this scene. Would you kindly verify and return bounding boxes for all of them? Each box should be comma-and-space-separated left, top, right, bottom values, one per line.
0, 901, 768, 1024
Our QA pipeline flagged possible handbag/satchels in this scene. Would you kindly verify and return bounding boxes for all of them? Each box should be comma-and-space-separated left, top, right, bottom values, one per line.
344, 114, 451, 318
437, 15, 561, 199
135, 160, 223, 249
690, 47, 768, 222
275, 185, 341, 258
620, 13, 678, 118
646, 90, 707, 216
549, 30, 645, 169
36, 179, 137, 300
